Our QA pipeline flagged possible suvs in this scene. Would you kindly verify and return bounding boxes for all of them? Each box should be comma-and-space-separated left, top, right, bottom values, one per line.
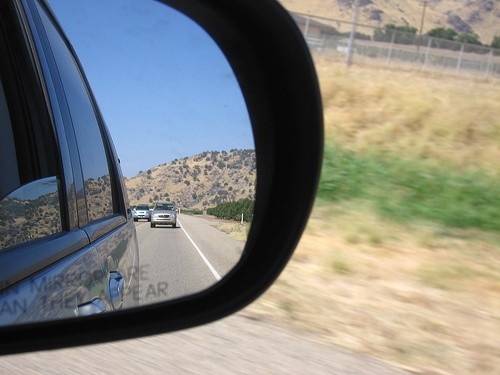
149, 202, 179, 228
132, 205, 151, 222
